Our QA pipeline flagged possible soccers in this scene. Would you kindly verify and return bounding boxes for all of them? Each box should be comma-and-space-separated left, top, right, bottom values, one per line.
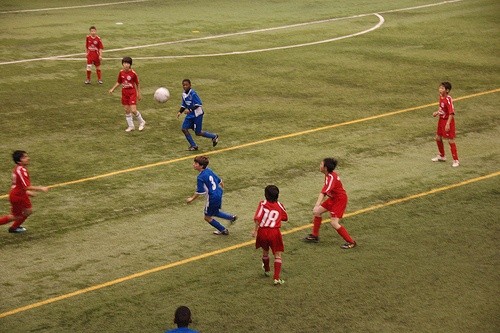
154, 87, 170, 102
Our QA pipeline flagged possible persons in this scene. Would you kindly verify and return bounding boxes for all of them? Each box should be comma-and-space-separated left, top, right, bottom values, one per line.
164, 306, 201, 333
252, 185, 288, 285
177, 79, 219, 151
0, 150, 49, 233
186, 155, 237, 235
433, 81, 460, 167
303, 157, 357, 249
109, 56, 146, 132
84, 26, 104, 85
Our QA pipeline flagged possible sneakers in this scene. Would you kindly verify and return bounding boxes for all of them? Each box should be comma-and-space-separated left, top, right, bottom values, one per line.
214, 229, 229, 236
213, 134, 219, 147
84, 80, 92, 84
342, 243, 357, 248
125, 125, 135, 132
433, 156, 447, 163
262, 263, 271, 276
274, 279, 286, 284
453, 159, 462, 169
303, 236, 318, 243
139, 119, 145, 131
97, 78, 103, 83
185, 145, 198, 151
228, 215, 237, 225
8, 227, 26, 232
